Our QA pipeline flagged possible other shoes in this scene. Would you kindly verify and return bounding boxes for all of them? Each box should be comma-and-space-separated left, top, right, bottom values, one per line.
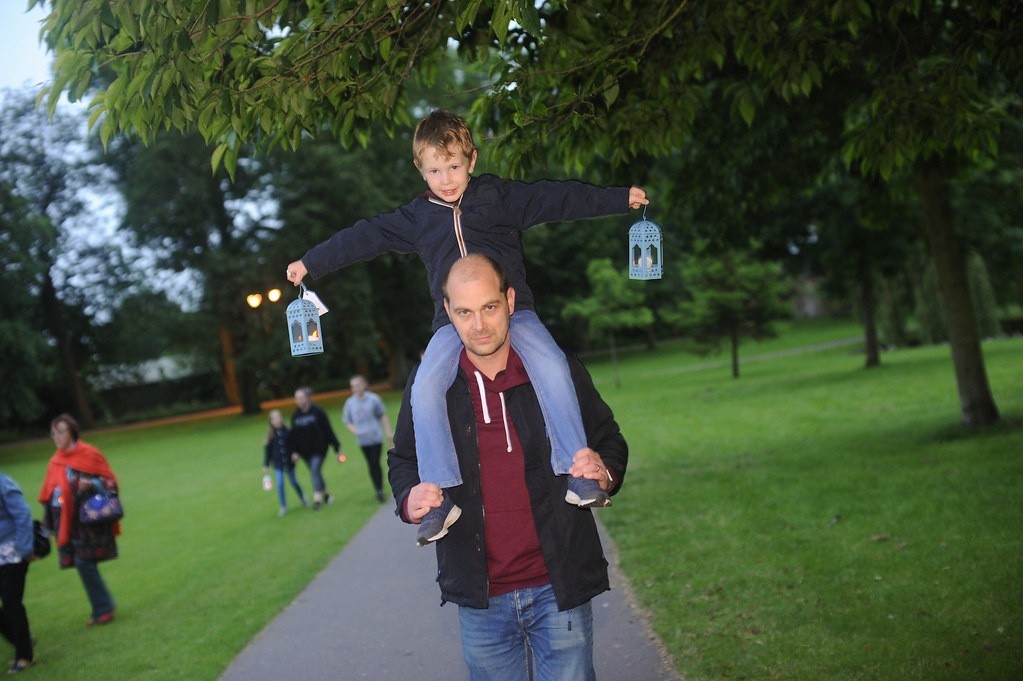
301, 497, 307, 510
376, 490, 385, 503
323, 494, 330, 504
85, 611, 113, 627
278, 506, 288, 517
313, 500, 320, 510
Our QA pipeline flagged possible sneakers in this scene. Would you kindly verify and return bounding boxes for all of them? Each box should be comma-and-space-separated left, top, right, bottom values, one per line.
565, 474, 612, 508
416, 492, 462, 547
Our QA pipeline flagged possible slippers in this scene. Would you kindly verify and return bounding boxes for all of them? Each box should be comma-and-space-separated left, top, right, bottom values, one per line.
7, 658, 35, 675
29, 633, 38, 647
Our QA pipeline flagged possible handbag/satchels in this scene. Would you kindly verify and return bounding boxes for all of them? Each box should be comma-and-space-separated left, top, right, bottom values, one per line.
28, 520, 51, 560
79, 479, 124, 523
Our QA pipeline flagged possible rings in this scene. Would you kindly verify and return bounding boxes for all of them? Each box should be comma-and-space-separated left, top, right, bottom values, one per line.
595, 464, 600, 472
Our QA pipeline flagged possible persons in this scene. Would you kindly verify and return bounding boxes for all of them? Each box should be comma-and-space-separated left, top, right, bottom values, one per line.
263, 375, 394, 517
287, 108, 650, 547
40, 414, 121, 625
0, 471, 34, 674
385, 252, 629, 681
